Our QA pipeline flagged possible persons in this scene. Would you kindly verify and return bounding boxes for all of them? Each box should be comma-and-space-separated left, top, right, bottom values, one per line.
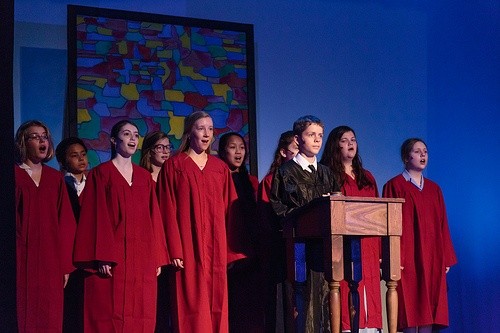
265, 115, 345, 280
72, 120, 172, 333
382, 137, 457, 333
139, 132, 174, 182
157, 111, 257, 333
259, 131, 300, 202
318, 126, 382, 333
218, 132, 266, 333
14, 120, 77, 333
55, 137, 89, 333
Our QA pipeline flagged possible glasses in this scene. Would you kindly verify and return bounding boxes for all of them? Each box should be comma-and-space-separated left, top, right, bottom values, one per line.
151, 143, 173, 153
25, 134, 48, 141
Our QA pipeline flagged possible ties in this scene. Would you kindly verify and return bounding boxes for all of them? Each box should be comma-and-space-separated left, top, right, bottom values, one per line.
308, 164, 317, 174
